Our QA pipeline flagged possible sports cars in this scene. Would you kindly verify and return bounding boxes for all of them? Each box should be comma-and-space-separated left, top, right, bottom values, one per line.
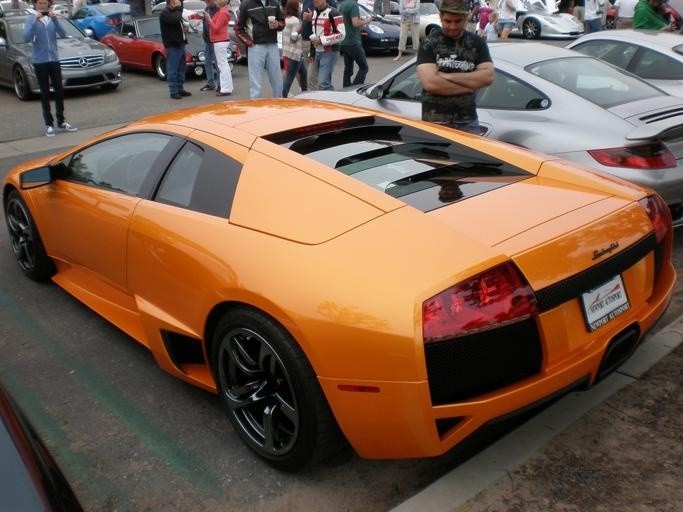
293, 39, 683, 235
0, 96, 676, 473
99, 14, 235, 81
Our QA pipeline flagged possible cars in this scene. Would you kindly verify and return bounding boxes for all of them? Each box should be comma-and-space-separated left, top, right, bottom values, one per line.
0, 0, 74, 19
358, 4, 412, 53
153, 0, 209, 26
562, 28, 682, 103
0, 15, 123, 100
227, 7, 284, 62
384, 2, 446, 43
517, 0, 585, 39
69, 2, 140, 42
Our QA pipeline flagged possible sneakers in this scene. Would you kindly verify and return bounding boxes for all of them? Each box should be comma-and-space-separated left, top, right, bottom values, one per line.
200, 85, 215, 91
46, 126, 55, 137
57, 123, 77, 131
171, 91, 190, 98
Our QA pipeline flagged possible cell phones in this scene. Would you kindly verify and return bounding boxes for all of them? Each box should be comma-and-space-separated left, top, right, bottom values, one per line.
41, 11, 49, 16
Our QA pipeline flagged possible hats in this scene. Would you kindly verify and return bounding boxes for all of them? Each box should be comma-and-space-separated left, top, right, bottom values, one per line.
439, 0, 470, 14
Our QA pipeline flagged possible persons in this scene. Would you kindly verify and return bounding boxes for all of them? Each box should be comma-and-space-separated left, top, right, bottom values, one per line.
394, 1, 517, 61
555, 1, 682, 35
418, 0, 495, 136
22, 2, 78, 136
160, 0, 372, 101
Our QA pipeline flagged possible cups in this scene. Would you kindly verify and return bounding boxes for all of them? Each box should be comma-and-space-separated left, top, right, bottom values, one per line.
266, 16, 276, 30
182, 22, 189, 32
290, 33, 298, 42
308, 34, 320, 49
